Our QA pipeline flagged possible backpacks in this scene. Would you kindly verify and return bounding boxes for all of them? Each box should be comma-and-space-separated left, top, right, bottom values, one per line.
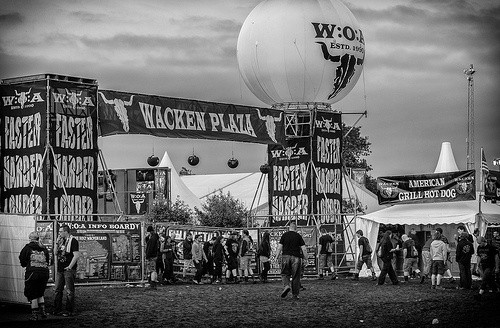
364, 242, 372, 254
409, 242, 418, 256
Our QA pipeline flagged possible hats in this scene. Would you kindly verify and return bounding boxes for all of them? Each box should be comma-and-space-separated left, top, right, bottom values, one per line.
147, 226, 154, 232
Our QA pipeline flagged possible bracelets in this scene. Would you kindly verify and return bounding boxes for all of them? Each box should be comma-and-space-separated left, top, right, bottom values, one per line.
56, 242, 59, 245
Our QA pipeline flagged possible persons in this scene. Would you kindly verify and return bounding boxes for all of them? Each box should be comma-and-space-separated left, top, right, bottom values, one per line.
146, 223, 500, 299
19, 232, 49, 320
47, 225, 80, 316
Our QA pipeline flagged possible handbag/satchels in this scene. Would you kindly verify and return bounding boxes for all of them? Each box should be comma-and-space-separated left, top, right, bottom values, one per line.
378, 246, 382, 257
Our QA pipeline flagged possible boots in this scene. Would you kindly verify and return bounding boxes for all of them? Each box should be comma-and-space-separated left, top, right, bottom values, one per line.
252, 274, 257, 284
372, 273, 377, 281
29, 308, 40, 321
350, 273, 359, 281
38, 302, 46, 317
226, 276, 248, 284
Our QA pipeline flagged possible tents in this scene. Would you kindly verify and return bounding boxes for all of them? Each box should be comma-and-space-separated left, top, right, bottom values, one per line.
160, 151, 209, 225
355, 201, 500, 278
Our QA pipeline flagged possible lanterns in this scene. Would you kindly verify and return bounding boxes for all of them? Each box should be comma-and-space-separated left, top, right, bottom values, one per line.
227, 158, 239, 168
147, 155, 159, 166
188, 155, 200, 166
260, 164, 271, 174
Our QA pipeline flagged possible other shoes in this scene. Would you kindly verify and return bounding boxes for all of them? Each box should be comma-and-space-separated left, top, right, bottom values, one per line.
420, 276, 425, 284
431, 285, 436, 289
280, 285, 291, 299
449, 277, 455, 283
53, 311, 62, 315
216, 280, 220, 282
193, 280, 198, 284
401, 280, 408, 283
331, 275, 337, 280
318, 278, 324, 280
436, 285, 446, 291
199, 282, 204, 284
62, 312, 73, 317
489, 290, 492, 292
479, 289, 484, 295
212, 281, 217, 284
293, 295, 300, 300
